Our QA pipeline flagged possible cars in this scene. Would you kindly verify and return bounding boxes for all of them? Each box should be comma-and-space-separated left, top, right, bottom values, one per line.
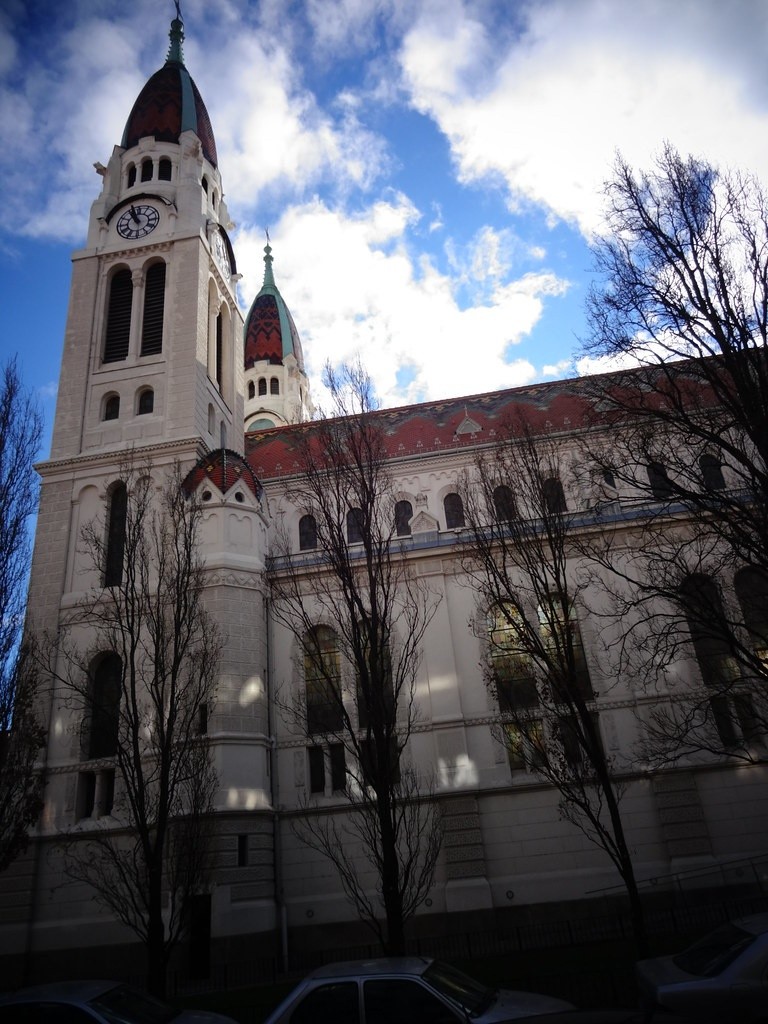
263, 955, 579, 1024
0, 978, 240, 1024
635, 909, 768, 1024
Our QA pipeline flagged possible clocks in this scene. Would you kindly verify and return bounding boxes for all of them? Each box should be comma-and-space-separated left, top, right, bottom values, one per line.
116, 204, 160, 239
